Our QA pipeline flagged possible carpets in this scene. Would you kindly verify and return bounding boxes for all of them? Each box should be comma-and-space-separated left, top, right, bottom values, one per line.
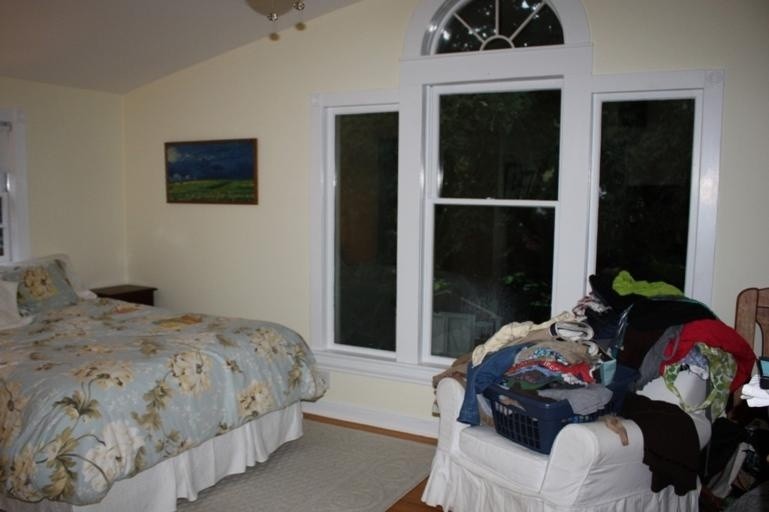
175, 419, 437, 512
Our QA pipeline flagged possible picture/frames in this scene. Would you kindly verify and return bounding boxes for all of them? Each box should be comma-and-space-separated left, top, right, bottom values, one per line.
164, 137, 258, 206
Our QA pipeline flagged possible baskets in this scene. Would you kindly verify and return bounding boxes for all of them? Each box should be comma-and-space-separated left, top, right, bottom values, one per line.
484, 383, 603, 458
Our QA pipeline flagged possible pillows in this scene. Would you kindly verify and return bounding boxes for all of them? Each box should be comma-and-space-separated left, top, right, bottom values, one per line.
0, 253, 98, 332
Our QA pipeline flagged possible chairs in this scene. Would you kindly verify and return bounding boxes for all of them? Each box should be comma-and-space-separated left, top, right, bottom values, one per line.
721, 287, 769, 412
435, 346, 713, 512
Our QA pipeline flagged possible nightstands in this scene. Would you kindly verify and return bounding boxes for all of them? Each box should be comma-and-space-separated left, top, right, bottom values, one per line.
90, 285, 157, 307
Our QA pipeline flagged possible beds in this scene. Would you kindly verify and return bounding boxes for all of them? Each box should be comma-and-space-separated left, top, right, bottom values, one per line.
0, 297, 302, 511
